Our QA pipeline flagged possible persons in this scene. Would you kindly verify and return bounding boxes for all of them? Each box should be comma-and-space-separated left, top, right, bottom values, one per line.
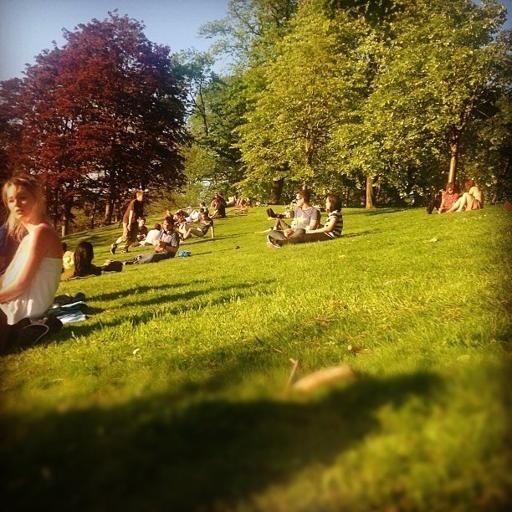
61, 241, 123, 277
0, 173, 64, 356
225, 194, 256, 207
110, 190, 227, 262
426, 180, 484, 214
262, 190, 342, 247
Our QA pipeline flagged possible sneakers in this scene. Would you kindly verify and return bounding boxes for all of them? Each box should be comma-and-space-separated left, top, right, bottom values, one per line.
267, 234, 282, 247
110, 243, 128, 254
